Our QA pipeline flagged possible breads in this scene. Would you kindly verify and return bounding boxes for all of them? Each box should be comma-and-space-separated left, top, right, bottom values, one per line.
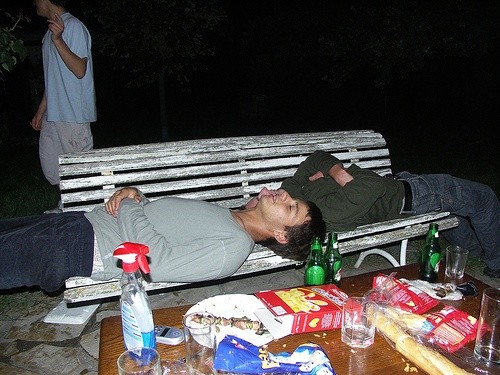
364, 304, 476, 375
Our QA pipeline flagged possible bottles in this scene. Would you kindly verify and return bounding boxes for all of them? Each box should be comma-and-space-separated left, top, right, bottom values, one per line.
113, 241, 156, 365
419, 223, 442, 283
304, 235, 326, 287
324, 232, 342, 290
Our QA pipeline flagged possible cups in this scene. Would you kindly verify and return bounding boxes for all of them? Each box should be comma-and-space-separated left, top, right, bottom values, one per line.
341, 296, 379, 349
473, 288, 500, 364
182, 311, 217, 375
117, 347, 160, 375
445, 246, 469, 279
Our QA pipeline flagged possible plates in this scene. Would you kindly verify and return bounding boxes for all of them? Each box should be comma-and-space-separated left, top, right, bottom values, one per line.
184, 294, 274, 350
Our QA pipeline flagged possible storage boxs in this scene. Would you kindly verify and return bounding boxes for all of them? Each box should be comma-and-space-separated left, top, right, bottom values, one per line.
252, 282, 361, 340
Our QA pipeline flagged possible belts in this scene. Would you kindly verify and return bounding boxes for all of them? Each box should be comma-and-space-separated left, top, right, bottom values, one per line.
387, 173, 413, 215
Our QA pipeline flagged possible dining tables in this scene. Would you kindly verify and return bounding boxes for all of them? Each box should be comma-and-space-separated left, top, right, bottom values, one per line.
98, 262, 500, 375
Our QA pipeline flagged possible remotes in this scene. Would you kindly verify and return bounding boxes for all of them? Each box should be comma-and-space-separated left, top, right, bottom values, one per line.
154, 326, 184, 345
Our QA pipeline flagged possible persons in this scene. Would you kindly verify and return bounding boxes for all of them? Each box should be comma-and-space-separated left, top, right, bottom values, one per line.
0, 187, 328, 294
245, 148, 500, 277
27, 0, 97, 203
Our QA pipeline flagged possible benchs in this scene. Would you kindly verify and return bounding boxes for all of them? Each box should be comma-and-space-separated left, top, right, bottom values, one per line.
58, 130, 460, 304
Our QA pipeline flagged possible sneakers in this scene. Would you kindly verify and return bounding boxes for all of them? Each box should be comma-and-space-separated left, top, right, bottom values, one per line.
43, 200, 63, 214
